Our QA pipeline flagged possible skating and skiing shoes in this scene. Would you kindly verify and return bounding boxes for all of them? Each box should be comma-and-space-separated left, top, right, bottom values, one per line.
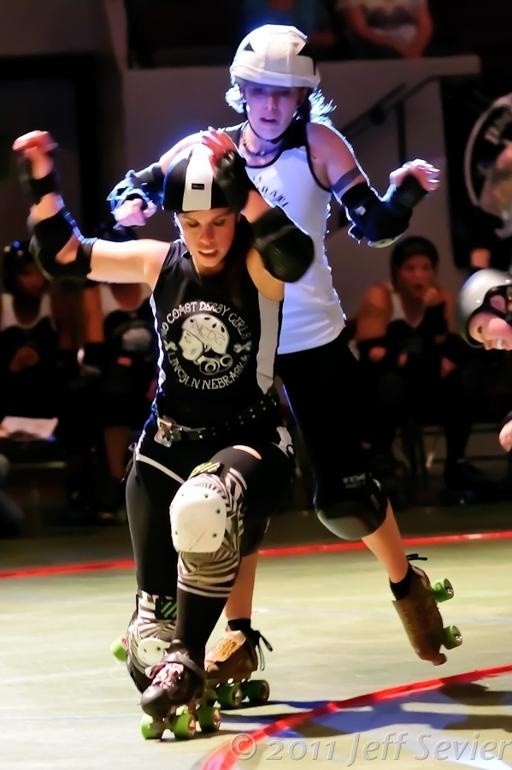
391, 563, 462, 667
140, 652, 222, 741
114, 588, 175, 696
203, 623, 274, 709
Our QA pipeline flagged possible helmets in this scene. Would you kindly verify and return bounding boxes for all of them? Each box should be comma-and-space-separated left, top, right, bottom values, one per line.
389, 234, 440, 285
0, 239, 49, 296
230, 23, 324, 102
160, 144, 243, 218
455, 263, 511, 349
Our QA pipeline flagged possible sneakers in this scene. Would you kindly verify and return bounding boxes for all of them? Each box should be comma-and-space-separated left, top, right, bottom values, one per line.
94, 475, 130, 523
409, 467, 477, 504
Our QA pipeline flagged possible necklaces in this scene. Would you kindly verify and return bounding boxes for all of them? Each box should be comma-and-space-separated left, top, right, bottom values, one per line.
242, 124, 282, 158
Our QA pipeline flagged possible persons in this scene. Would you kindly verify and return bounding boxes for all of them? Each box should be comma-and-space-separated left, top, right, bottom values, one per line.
10, 124, 311, 718
2, 237, 510, 530
108, 24, 442, 677
